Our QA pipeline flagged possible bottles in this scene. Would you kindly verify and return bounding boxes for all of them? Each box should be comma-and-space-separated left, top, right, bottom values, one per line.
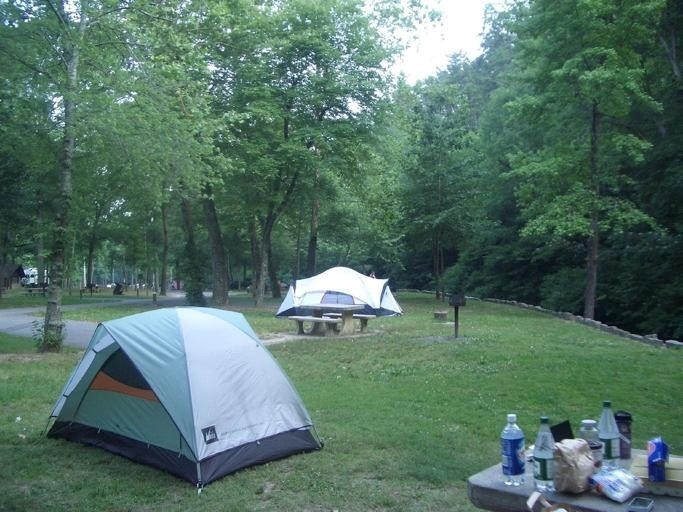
599, 399, 621, 471
532, 416, 556, 492
579, 419, 603, 475
500, 413, 526, 487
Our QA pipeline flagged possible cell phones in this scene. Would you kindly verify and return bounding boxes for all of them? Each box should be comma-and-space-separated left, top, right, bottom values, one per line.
627, 496, 656, 512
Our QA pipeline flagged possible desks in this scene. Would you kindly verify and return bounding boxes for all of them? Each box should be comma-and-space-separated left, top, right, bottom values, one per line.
466, 438, 683, 511
294, 303, 365, 334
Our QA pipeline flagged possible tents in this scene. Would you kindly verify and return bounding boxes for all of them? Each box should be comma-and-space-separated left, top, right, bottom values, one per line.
273, 264, 405, 321
38, 304, 326, 492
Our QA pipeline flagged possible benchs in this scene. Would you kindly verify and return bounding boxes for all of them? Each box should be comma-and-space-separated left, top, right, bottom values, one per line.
287, 312, 376, 339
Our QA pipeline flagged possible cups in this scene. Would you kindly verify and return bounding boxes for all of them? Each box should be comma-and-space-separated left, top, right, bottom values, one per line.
614, 408, 633, 460
